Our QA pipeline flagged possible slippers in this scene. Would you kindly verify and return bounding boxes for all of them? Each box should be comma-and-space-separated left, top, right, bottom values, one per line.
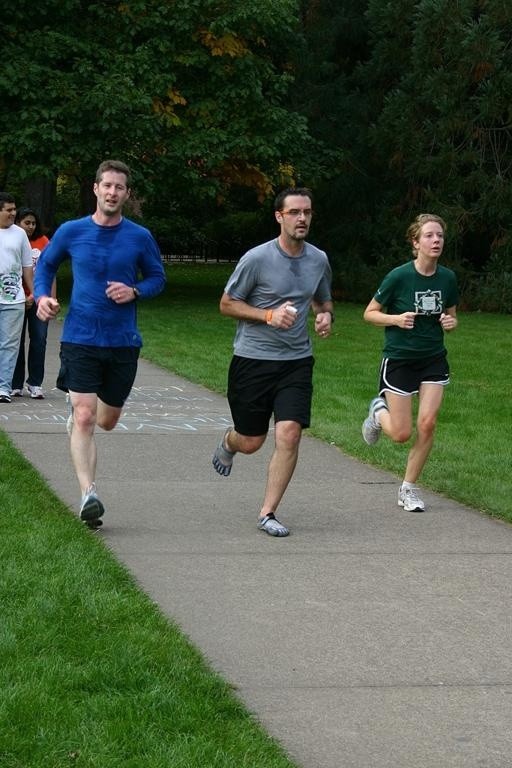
212, 427, 235, 476
257, 511, 290, 536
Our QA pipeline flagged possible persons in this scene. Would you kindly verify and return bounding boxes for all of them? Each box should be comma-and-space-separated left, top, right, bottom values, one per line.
32, 159, 167, 530
211, 186, 336, 538
12, 206, 57, 399
0, 192, 34, 403
360, 212, 459, 513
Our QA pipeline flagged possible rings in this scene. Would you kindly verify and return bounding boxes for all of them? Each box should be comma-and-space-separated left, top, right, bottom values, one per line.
117, 294, 121, 299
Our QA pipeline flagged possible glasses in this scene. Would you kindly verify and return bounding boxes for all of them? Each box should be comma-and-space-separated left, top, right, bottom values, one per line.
281, 209, 312, 215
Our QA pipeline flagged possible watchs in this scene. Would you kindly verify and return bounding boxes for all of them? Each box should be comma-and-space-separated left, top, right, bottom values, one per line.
132, 285, 141, 299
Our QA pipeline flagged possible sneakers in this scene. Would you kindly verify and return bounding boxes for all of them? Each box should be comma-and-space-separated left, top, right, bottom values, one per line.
398, 487, 425, 511
66, 393, 74, 434
0, 385, 44, 402
79, 492, 105, 527
362, 398, 387, 445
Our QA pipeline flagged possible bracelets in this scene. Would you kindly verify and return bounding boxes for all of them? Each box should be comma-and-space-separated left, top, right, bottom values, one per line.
327, 309, 335, 322
266, 308, 273, 325
